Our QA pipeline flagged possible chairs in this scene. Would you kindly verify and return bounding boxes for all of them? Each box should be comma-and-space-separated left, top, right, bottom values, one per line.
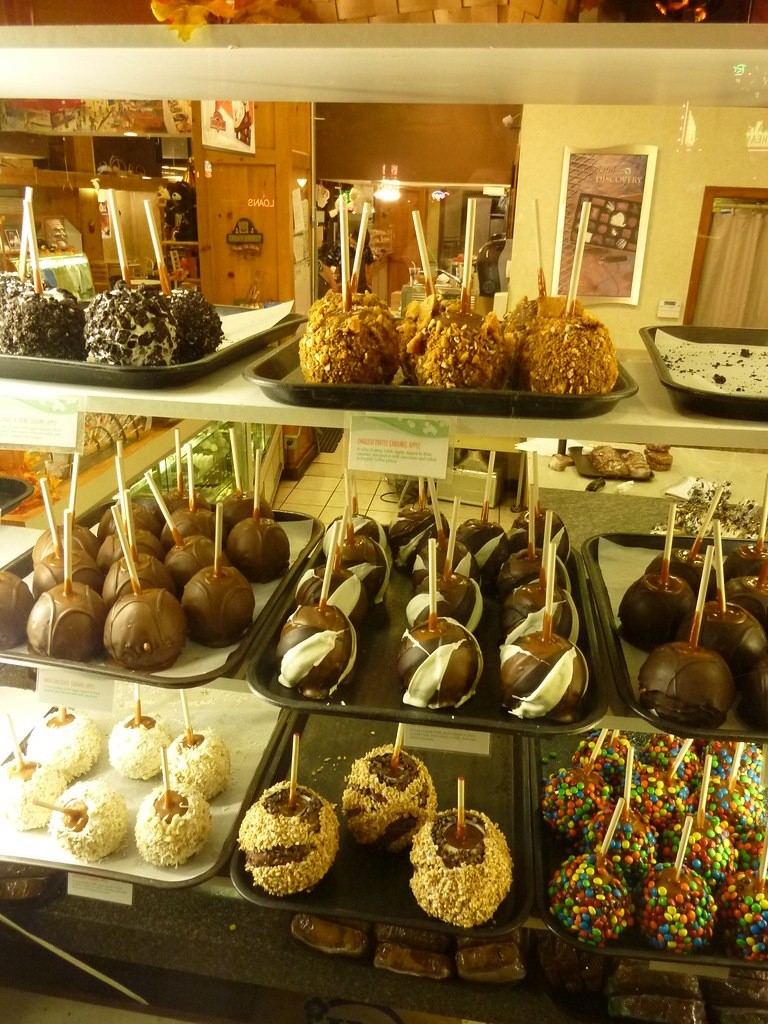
170, 249, 201, 290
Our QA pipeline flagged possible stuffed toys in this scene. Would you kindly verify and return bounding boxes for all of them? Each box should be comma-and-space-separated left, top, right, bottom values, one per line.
165, 181, 195, 227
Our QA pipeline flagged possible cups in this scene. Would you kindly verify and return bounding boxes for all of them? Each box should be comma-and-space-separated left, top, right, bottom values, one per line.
408, 267, 420, 285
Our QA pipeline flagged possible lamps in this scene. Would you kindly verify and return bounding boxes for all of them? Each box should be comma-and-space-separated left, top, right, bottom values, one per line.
372, 162, 403, 201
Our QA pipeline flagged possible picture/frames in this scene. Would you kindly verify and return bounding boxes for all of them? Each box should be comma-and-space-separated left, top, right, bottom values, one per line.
552, 143, 658, 308
97, 200, 112, 238
202, 99, 256, 155
5, 229, 20, 250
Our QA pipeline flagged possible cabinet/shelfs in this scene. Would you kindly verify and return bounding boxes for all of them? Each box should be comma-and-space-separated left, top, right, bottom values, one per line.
366, 254, 395, 307
0, 340, 768, 1024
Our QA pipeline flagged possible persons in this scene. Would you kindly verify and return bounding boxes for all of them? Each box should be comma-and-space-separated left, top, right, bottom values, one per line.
328, 229, 373, 293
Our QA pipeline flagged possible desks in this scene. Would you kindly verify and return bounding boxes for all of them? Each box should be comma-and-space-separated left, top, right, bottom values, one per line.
112, 263, 141, 278
11, 253, 94, 304
131, 278, 160, 286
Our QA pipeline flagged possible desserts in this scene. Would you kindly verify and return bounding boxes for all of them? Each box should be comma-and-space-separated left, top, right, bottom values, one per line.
0, 448, 768, 1024
297, 290, 618, 396
0, 268, 225, 369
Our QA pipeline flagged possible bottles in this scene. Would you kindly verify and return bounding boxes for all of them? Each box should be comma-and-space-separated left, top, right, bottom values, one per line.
451, 254, 464, 283
